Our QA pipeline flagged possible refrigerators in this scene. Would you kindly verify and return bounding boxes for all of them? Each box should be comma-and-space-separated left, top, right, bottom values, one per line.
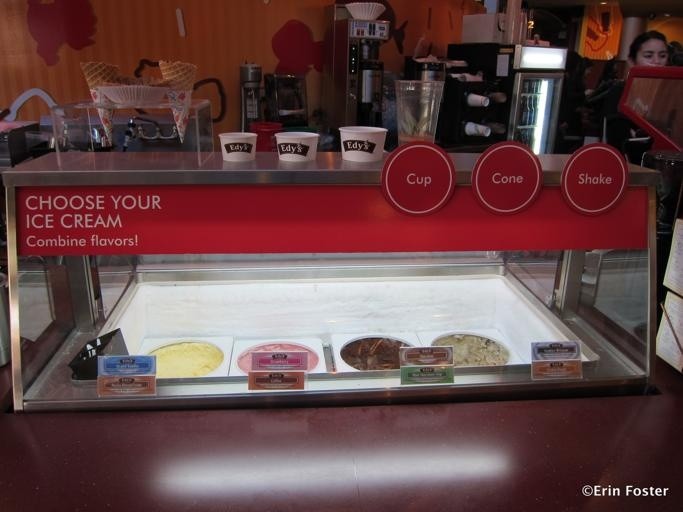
446, 45, 567, 155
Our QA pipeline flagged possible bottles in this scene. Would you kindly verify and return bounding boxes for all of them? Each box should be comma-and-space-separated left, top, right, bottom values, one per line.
527, 96, 535, 125
524, 129, 531, 144
522, 81, 529, 93
517, 129, 524, 142
519, 95, 529, 125
532, 82, 538, 93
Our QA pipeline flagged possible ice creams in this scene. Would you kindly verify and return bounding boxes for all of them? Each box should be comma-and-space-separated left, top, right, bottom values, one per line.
159, 60, 196, 144
81, 63, 120, 145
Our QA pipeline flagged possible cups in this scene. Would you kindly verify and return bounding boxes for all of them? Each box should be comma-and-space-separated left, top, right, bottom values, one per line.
394, 55, 446, 146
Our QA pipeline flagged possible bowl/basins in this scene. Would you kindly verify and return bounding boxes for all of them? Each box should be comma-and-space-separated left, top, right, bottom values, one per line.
339, 126, 389, 162
275, 132, 319, 162
345, 2, 387, 20
219, 132, 258, 161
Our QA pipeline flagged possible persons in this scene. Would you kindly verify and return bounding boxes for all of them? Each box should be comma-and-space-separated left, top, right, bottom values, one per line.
559, 31, 677, 168
